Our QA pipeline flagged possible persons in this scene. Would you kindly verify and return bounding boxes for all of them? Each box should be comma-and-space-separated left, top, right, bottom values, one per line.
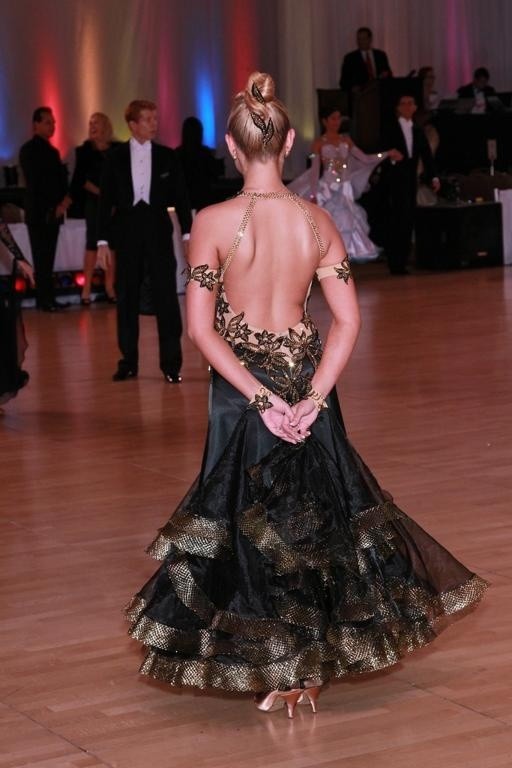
0, 189, 37, 405
455, 65, 500, 112
169, 115, 225, 218
16, 107, 68, 311
68, 111, 128, 306
417, 66, 443, 110
306, 103, 404, 269
375, 93, 442, 275
338, 26, 393, 120
182, 71, 364, 716
93, 100, 195, 382
411, 101, 442, 187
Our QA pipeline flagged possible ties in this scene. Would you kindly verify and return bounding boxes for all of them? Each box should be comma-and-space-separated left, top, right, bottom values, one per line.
365, 53, 375, 78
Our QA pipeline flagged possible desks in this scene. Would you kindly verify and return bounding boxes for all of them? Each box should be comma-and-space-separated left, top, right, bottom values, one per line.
0, 219, 102, 299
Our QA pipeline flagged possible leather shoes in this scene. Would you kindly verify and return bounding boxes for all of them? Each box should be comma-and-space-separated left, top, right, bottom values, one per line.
112, 367, 138, 382
37, 301, 59, 311
164, 373, 182, 383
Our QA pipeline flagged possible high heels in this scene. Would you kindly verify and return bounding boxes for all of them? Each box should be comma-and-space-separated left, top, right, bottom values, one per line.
256, 691, 300, 719
297, 686, 319, 713
81, 287, 90, 305
104, 292, 117, 304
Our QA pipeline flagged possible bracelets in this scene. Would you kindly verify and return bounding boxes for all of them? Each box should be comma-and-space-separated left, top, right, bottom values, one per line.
305, 386, 328, 410
246, 387, 273, 414
